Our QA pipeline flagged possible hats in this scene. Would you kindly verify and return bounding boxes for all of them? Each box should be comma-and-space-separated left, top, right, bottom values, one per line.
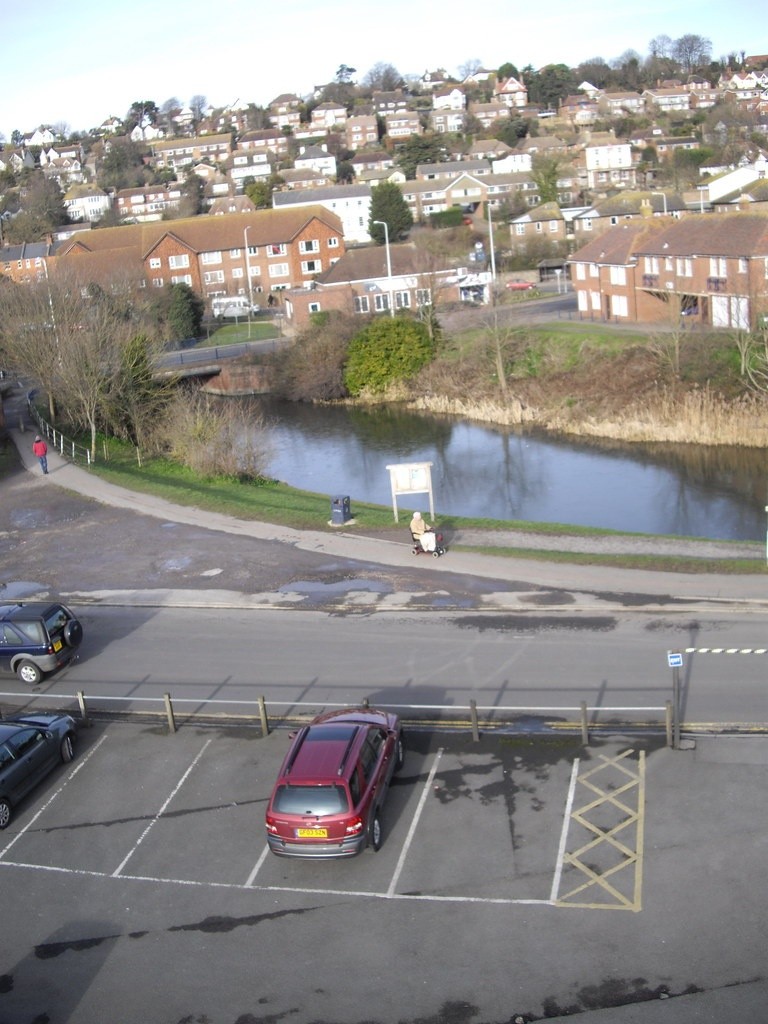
35, 435, 40, 439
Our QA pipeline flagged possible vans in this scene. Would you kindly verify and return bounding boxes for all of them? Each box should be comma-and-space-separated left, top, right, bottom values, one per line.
212, 296, 260, 322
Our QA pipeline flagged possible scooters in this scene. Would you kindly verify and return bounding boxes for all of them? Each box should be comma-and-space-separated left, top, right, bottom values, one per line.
410, 529, 446, 558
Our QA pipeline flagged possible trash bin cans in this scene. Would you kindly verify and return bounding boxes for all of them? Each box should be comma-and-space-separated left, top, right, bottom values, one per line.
331, 494, 350, 524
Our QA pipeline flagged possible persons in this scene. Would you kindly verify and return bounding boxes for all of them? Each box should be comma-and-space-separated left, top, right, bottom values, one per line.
33, 436, 48, 473
411, 512, 435, 551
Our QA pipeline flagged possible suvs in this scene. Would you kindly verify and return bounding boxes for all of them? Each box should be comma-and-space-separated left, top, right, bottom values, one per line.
0, 603, 84, 684
265, 707, 404, 859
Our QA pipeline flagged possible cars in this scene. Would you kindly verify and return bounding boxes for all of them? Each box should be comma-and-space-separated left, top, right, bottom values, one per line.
0, 711, 79, 830
506, 278, 536, 290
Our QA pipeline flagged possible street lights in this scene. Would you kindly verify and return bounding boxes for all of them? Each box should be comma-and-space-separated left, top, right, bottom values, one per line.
243, 225, 255, 315
36, 256, 62, 365
697, 188, 705, 213
487, 204, 496, 280
651, 191, 667, 215
373, 220, 395, 316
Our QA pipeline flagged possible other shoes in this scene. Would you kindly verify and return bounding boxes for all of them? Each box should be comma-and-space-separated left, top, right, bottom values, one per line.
43, 470, 49, 474
